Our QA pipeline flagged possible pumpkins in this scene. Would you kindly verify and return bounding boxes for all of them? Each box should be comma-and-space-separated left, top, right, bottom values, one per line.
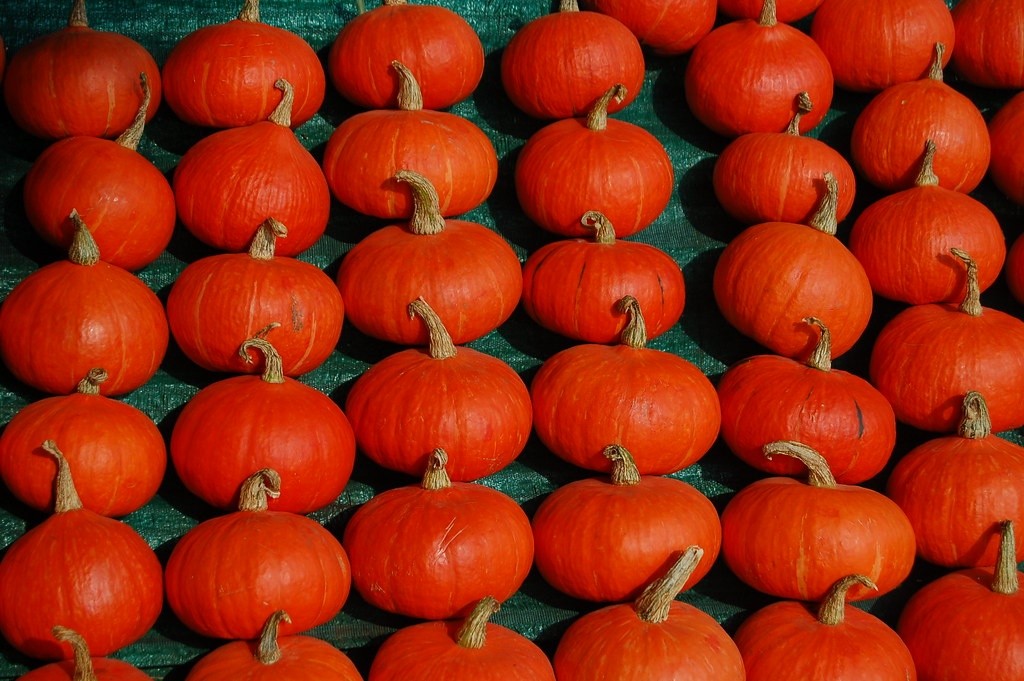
1, 0, 1024, 680
